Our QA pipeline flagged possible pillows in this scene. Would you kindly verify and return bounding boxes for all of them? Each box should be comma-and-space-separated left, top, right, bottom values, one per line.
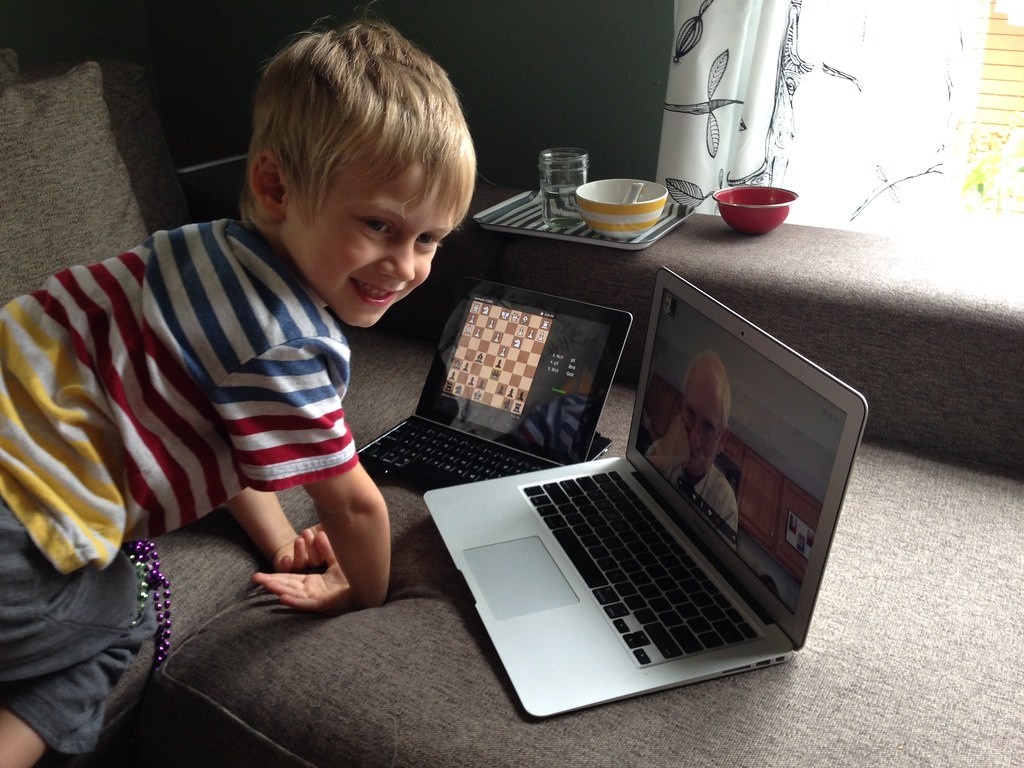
0, 61, 149, 307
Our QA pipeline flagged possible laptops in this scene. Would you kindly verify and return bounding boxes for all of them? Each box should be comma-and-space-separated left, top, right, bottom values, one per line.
422, 265, 867, 717
357, 278, 633, 494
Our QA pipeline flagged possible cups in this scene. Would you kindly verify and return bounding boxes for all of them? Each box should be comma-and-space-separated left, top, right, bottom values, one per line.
539, 148, 589, 228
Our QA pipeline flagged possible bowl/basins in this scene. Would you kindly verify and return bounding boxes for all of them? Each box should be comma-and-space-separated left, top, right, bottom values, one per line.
575, 179, 668, 239
713, 186, 799, 233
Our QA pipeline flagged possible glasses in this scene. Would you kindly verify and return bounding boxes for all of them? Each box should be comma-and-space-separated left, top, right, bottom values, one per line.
682, 405, 724, 443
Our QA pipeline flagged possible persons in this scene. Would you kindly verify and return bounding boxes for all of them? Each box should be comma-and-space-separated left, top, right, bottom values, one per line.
0, 21, 476, 768
643, 351, 740, 552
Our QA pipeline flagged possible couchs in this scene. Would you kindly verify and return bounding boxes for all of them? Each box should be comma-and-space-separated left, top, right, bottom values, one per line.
0, 60, 437, 768
129, 386, 1024, 768
